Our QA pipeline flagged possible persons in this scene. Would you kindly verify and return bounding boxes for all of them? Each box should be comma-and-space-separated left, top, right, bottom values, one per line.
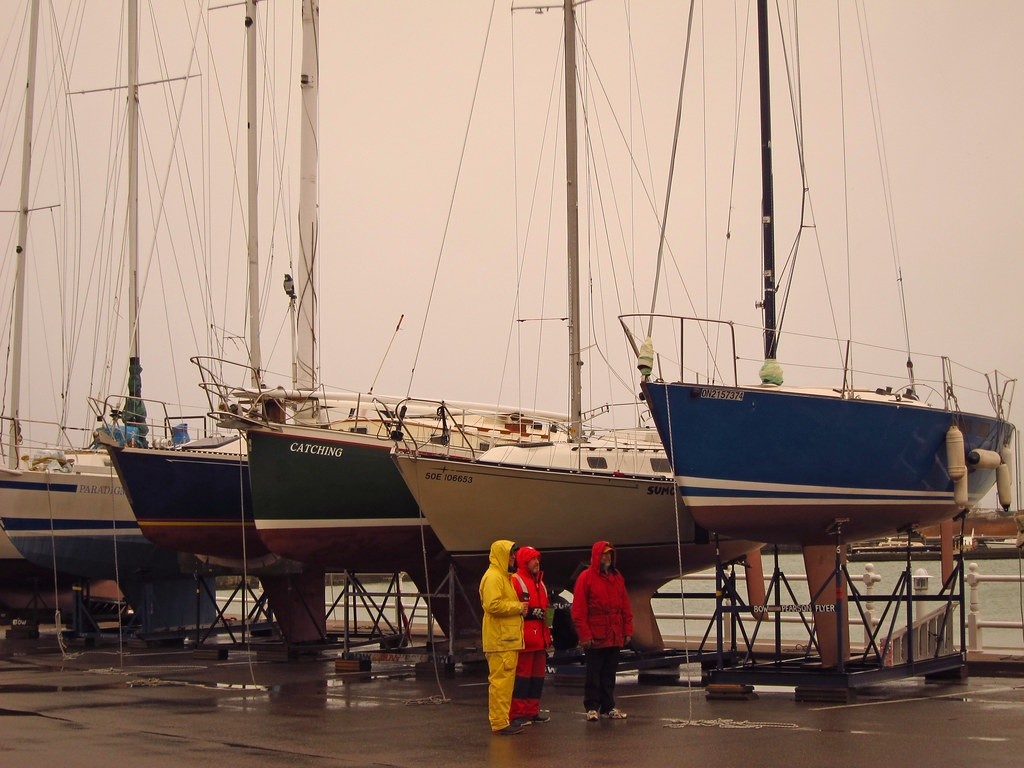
511, 546, 555, 726
571, 541, 635, 721
479, 540, 529, 735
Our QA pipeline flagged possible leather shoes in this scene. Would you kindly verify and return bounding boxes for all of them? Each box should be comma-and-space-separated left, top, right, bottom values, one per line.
510, 720, 522, 726
532, 715, 551, 723
518, 716, 531, 726
493, 726, 525, 735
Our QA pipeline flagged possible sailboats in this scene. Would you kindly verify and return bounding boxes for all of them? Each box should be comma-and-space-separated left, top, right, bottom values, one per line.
2, 1, 229, 577
84, 2, 321, 564
618, 2, 1018, 546
389, 0, 720, 592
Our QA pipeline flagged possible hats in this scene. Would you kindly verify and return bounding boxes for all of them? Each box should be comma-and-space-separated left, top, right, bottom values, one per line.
602, 544, 615, 554
511, 543, 520, 550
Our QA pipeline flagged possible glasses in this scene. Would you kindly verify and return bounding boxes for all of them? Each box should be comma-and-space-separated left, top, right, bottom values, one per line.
510, 551, 516, 556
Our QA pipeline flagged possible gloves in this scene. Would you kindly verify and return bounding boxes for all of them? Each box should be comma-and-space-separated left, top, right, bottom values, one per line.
551, 602, 572, 609
527, 608, 545, 620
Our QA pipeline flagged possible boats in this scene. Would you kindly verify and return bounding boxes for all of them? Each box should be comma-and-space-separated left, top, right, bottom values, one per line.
190, 356, 578, 569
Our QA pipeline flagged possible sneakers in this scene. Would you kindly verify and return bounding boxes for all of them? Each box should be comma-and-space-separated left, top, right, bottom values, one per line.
601, 708, 627, 720
586, 710, 599, 722
